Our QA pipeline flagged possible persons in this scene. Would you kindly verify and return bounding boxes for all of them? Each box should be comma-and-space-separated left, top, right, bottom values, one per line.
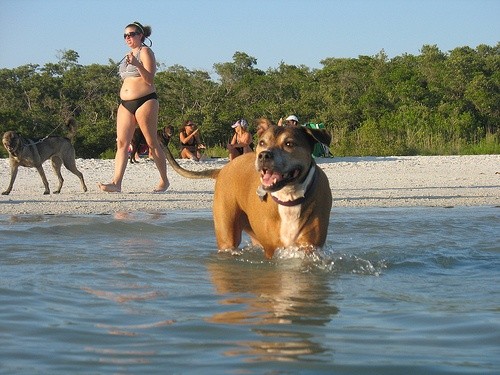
278, 115, 299, 126
96, 22, 170, 193
227, 120, 255, 162
127, 143, 143, 161
179, 120, 207, 161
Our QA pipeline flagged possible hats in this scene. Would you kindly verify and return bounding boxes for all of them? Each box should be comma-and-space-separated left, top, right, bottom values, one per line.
231, 119, 248, 129
285, 115, 299, 123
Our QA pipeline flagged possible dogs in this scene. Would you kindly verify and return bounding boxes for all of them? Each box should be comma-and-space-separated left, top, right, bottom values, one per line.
130, 125, 175, 163
0, 116, 88, 195
163, 117, 332, 260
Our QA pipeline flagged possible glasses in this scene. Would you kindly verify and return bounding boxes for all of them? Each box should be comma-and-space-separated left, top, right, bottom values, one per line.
185, 123, 194, 126
124, 31, 140, 38
